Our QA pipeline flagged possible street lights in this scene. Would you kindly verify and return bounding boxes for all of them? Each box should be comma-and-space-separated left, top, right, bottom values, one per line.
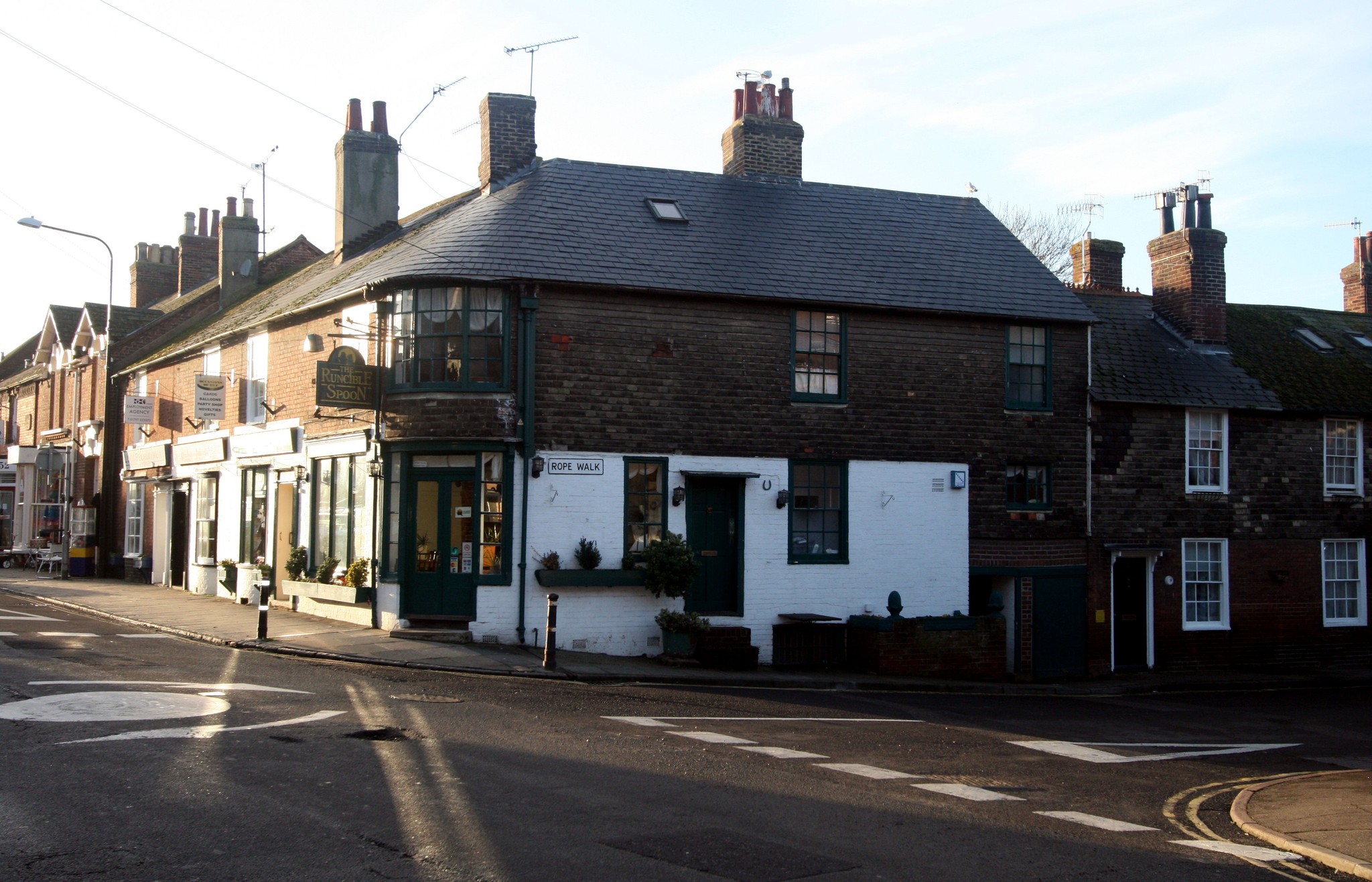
17, 217, 114, 577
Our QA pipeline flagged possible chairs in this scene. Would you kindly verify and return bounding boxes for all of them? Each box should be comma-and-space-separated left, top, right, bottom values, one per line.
35, 544, 72, 579
418, 550, 437, 572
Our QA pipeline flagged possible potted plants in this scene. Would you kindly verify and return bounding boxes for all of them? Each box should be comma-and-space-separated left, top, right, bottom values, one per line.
216, 559, 236, 570
530, 530, 702, 600
654, 608, 712, 657
110, 552, 124, 558
251, 563, 272, 574
281, 545, 371, 604
133, 553, 153, 561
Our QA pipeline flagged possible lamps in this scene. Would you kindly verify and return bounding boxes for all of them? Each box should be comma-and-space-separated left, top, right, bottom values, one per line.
673, 486, 685, 506
292, 464, 309, 482
367, 459, 385, 480
777, 489, 791, 509
532, 455, 545, 479
303, 334, 317, 352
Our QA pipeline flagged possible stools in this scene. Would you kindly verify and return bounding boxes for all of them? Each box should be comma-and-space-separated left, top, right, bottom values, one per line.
23, 543, 39, 571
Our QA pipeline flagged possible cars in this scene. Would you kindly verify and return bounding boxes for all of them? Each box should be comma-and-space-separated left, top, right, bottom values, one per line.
0, 551, 18, 569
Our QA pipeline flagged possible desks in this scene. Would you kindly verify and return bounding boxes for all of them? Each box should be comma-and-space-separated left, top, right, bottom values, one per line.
778, 614, 842, 623
